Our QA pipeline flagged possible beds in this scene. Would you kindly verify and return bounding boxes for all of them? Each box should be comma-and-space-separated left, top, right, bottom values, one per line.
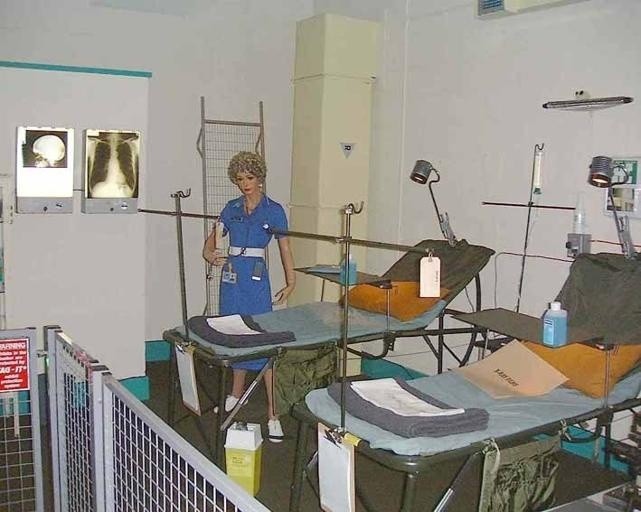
292, 253, 641, 512
163, 239, 495, 469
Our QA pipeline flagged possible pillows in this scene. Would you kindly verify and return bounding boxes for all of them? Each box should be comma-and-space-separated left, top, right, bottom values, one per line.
339, 278, 452, 322
522, 329, 641, 399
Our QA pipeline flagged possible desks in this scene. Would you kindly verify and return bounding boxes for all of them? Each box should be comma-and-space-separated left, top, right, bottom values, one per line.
450, 309, 614, 410
292, 265, 392, 332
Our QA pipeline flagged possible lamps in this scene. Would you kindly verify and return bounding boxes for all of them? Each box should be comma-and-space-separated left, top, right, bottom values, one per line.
409, 160, 457, 246
588, 156, 635, 259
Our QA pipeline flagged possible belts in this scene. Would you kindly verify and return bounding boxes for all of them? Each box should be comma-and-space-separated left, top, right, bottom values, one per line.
227, 246, 265, 258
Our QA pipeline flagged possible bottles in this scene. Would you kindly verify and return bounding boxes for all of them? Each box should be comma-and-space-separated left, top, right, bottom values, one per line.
542, 301, 569, 348
341, 253, 358, 283
572, 191, 586, 234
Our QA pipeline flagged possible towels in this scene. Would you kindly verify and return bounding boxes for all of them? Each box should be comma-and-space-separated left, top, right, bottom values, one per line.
187, 314, 296, 348
328, 376, 490, 437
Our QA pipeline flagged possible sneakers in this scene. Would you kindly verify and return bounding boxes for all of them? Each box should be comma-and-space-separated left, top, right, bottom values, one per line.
214, 395, 248, 414
268, 420, 284, 443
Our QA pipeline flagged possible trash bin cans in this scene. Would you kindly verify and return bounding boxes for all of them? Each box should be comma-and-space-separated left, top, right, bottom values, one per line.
224, 422, 264, 498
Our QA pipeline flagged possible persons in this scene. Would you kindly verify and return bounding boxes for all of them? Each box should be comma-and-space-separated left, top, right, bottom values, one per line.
202, 151, 296, 444
